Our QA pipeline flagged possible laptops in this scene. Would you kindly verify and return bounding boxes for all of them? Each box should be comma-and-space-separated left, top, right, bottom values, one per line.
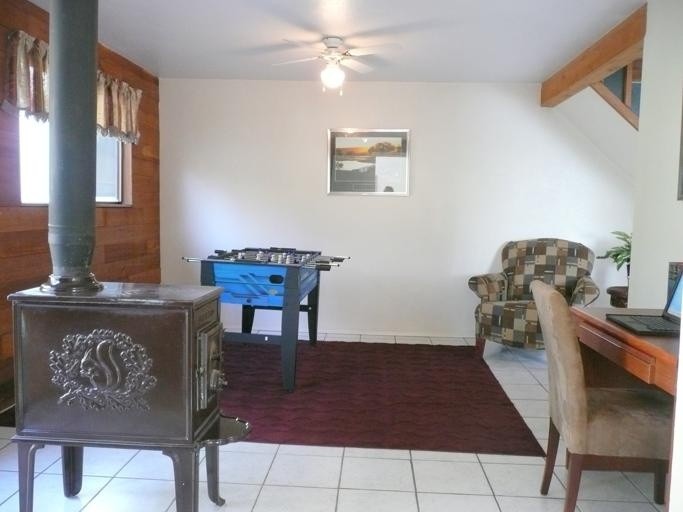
606, 269, 683, 337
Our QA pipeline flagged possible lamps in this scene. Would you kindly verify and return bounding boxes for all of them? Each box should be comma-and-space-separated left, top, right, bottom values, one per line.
320, 36, 344, 99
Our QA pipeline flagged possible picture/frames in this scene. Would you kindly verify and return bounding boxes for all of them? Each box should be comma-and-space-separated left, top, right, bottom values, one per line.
326, 127, 411, 198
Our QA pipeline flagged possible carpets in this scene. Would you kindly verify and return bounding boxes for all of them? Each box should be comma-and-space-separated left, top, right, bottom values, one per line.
213, 335, 547, 458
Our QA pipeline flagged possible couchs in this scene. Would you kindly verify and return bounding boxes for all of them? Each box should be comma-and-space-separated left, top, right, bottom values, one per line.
469, 238, 599, 364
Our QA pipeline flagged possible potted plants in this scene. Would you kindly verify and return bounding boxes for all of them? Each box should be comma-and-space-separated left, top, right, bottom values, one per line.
596, 230, 633, 280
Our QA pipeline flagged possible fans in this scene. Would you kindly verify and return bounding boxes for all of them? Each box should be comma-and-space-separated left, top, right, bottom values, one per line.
270, 31, 395, 75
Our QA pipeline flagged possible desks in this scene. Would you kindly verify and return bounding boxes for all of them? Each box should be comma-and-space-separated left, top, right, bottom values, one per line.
569, 304, 678, 398
606, 284, 628, 308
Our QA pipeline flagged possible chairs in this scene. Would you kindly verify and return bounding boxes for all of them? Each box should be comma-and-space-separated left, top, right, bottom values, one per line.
528, 278, 674, 512
